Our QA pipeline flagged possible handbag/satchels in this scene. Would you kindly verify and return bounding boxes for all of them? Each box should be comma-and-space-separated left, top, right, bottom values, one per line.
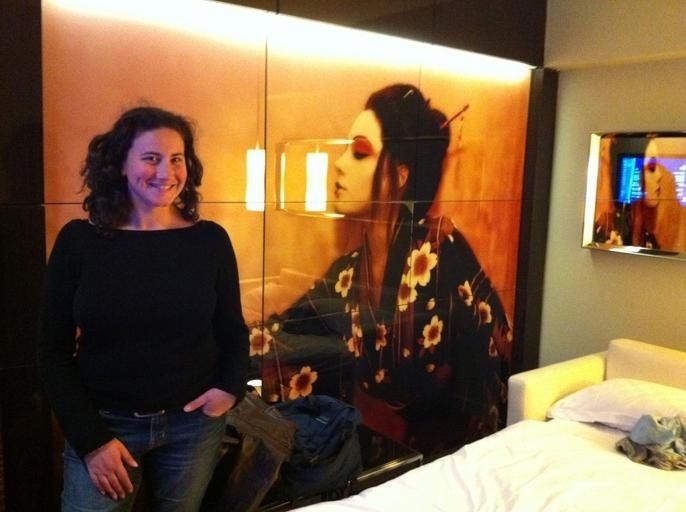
275, 394, 359, 500
218, 392, 299, 511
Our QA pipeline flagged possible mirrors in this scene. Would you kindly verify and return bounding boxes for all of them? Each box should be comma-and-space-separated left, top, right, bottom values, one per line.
582, 127, 686, 261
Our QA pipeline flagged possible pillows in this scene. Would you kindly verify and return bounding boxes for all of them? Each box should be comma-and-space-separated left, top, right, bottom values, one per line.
546, 373, 686, 440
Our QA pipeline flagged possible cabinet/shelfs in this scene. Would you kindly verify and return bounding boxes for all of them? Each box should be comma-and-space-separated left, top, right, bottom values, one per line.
263, 422, 426, 511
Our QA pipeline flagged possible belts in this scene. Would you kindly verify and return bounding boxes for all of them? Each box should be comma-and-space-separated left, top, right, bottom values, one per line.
102, 408, 171, 418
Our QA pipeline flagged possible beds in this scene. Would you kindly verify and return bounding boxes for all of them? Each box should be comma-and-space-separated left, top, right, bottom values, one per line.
279, 418, 686, 507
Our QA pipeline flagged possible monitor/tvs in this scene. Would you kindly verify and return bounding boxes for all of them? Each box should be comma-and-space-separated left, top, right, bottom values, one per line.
614, 153, 686, 210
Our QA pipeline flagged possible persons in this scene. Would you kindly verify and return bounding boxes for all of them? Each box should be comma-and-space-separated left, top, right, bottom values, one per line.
36, 108, 250, 509
244, 83, 512, 464
594, 133, 661, 249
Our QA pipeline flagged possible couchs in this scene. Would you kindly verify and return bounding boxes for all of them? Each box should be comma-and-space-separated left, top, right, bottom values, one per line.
506, 338, 686, 475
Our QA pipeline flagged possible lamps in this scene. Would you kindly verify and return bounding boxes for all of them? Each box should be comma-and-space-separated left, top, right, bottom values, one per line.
246, 41, 265, 213
274, 139, 352, 220
